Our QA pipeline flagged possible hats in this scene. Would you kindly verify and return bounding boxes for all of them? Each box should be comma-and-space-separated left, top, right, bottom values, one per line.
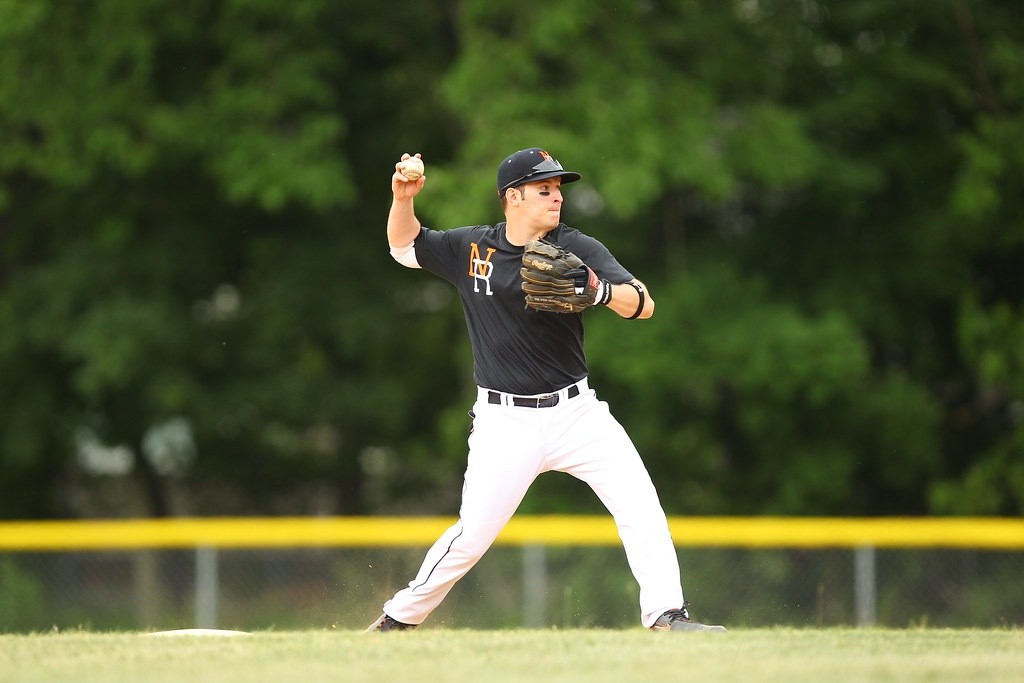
496, 147, 583, 197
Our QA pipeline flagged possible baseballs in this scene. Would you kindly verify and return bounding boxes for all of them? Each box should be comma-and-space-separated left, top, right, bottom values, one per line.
401, 157, 424, 182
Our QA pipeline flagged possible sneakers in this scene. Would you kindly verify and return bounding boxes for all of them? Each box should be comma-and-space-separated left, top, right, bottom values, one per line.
362, 611, 418, 632
651, 600, 727, 633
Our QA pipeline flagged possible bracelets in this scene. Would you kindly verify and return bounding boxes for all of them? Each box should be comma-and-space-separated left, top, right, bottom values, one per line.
621, 282, 644, 320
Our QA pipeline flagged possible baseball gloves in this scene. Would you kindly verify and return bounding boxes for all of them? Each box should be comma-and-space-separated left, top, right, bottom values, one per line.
519, 237, 599, 316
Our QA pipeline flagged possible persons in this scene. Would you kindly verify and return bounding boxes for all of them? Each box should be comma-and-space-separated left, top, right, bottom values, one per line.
364, 148, 728, 634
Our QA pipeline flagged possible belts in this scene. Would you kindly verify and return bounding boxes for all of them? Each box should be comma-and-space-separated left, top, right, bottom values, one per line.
488, 384, 579, 409
